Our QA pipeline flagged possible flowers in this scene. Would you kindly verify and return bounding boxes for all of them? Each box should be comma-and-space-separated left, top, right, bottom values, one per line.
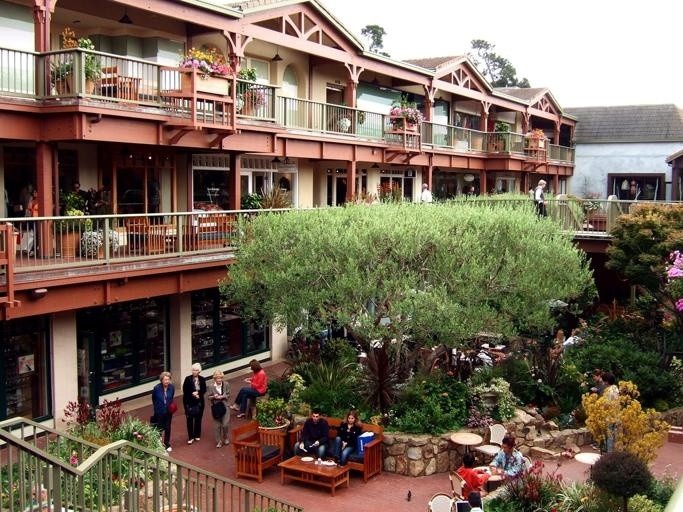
254, 396, 287, 427
389, 93, 424, 128
236, 67, 266, 109
0, 398, 171, 512
176, 45, 230, 81
80, 228, 118, 257
527, 128, 545, 140
581, 190, 603, 211
52, 209, 83, 233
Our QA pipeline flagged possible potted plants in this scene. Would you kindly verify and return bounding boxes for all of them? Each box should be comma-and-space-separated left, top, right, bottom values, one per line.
49, 57, 100, 96
236, 96, 256, 120
492, 122, 509, 152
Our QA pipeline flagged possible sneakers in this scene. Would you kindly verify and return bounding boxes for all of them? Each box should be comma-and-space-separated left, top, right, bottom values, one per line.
188, 439, 195, 444
216, 441, 222, 448
230, 405, 240, 412
166, 447, 172, 452
224, 439, 230, 445
236, 413, 245, 418
195, 437, 200, 441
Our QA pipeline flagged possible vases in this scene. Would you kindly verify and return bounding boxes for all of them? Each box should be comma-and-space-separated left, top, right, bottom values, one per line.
59, 232, 78, 257
96, 244, 104, 259
257, 418, 289, 435
585, 208, 597, 218
179, 73, 229, 98
530, 140, 544, 148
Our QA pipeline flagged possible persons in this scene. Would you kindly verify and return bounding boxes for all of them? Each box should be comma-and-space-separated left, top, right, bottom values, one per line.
590, 369, 610, 450
528, 186, 534, 195
465, 490, 486, 512
533, 178, 549, 217
465, 185, 477, 196
292, 315, 583, 384
65, 182, 91, 204
152, 358, 267, 451
420, 183, 435, 202
599, 371, 621, 452
294, 408, 362, 466
457, 437, 521, 500
24, 187, 37, 215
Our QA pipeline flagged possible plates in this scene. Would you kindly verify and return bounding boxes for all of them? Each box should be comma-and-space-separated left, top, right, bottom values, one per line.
300, 456, 314, 461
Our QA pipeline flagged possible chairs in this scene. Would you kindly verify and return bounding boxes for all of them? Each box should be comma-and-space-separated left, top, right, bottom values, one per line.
231, 420, 287, 483
427, 423, 532, 512
101, 66, 131, 99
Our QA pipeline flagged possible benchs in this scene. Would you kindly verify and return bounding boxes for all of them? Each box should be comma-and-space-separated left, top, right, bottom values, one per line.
288, 417, 383, 482
180, 216, 235, 251
123, 217, 157, 256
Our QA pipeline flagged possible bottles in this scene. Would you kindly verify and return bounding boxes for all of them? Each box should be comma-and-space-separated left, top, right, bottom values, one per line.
317, 458, 321, 465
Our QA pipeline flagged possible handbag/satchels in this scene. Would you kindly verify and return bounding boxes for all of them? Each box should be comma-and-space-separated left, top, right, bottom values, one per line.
167, 399, 178, 415
211, 402, 226, 420
186, 405, 200, 416
150, 415, 165, 430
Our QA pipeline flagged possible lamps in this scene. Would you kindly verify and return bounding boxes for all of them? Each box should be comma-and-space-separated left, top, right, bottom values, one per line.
269, 156, 281, 163
370, 163, 380, 168
619, 177, 631, 191
